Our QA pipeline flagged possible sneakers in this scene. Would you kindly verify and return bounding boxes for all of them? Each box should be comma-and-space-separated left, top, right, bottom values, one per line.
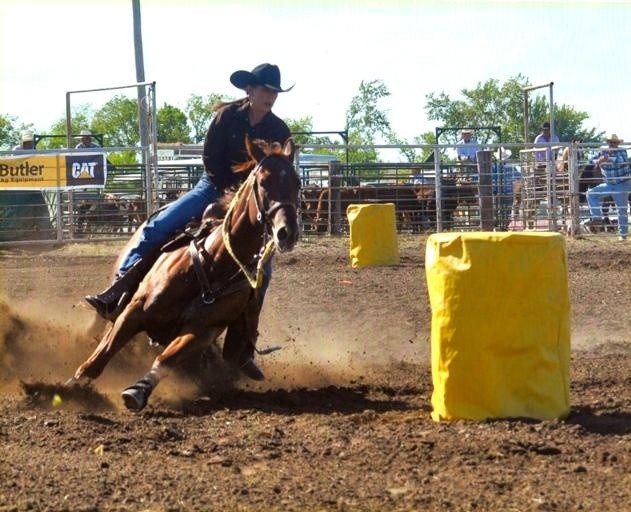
619, 236, 628, 241
584, 218, 602, 226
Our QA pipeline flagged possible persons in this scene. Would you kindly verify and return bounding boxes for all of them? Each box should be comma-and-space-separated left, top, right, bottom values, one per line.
82, 60, 296, 383
465, 145, 522, 233
455, 131, 483, 179
585, 133, 631, 242
563, 138, 578, 171
531, 122, 564, 166
406, 165, 428, 184
73, 129, 101, 196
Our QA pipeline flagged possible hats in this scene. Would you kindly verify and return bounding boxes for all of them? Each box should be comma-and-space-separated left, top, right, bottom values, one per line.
73, 130, 93, 139
461, 125, 473, 134
540, 122, 550, 131
21, 130, 34, 143
229, 62, 296, 93
603, 134, 624, 143
494, 146, 512, 161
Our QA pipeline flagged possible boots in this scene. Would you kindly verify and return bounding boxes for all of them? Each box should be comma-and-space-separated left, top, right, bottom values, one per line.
85, 274, 127, 315
222, 324, 266, 382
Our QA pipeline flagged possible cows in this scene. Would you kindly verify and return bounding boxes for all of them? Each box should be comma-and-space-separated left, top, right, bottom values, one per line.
300, 182, 476, 238
75, 176, 188, 236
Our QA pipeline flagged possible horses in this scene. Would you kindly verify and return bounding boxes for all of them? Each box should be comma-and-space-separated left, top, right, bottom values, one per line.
457, 154, 522, 230
61, 131, 303, 414
513, 137, 631, 229
